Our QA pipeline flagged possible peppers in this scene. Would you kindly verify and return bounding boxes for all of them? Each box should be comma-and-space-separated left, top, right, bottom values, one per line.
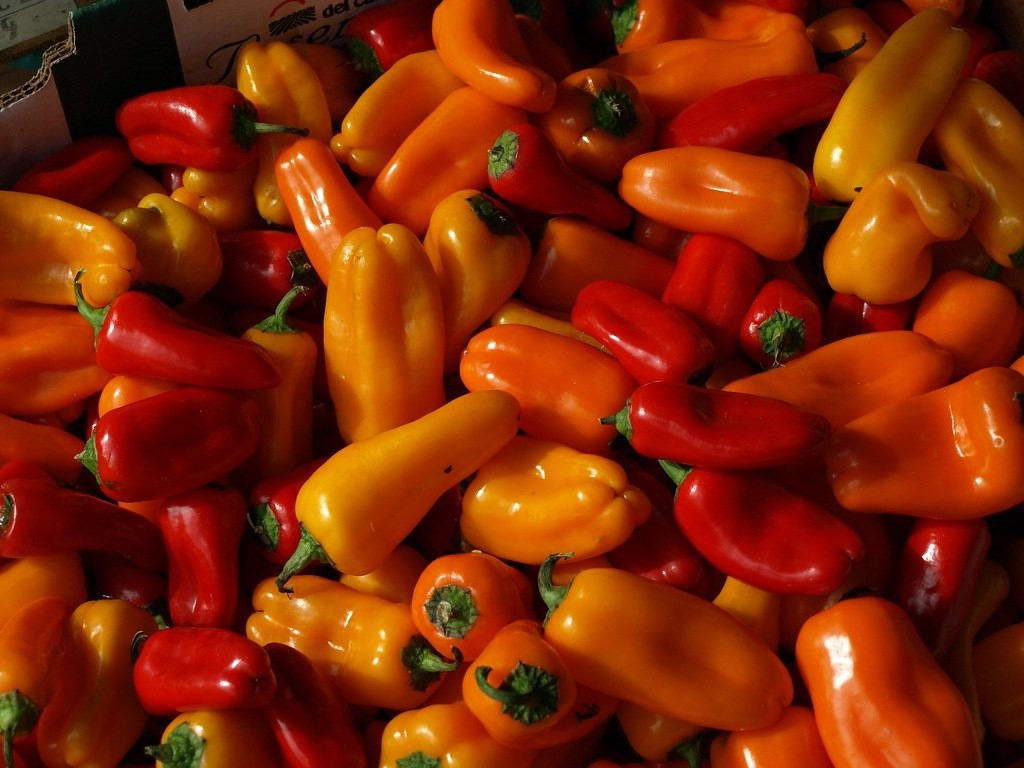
0, 0, 1024, 768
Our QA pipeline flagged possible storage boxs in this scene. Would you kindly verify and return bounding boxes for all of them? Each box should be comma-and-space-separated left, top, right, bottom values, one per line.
0, 0, 389, 180
